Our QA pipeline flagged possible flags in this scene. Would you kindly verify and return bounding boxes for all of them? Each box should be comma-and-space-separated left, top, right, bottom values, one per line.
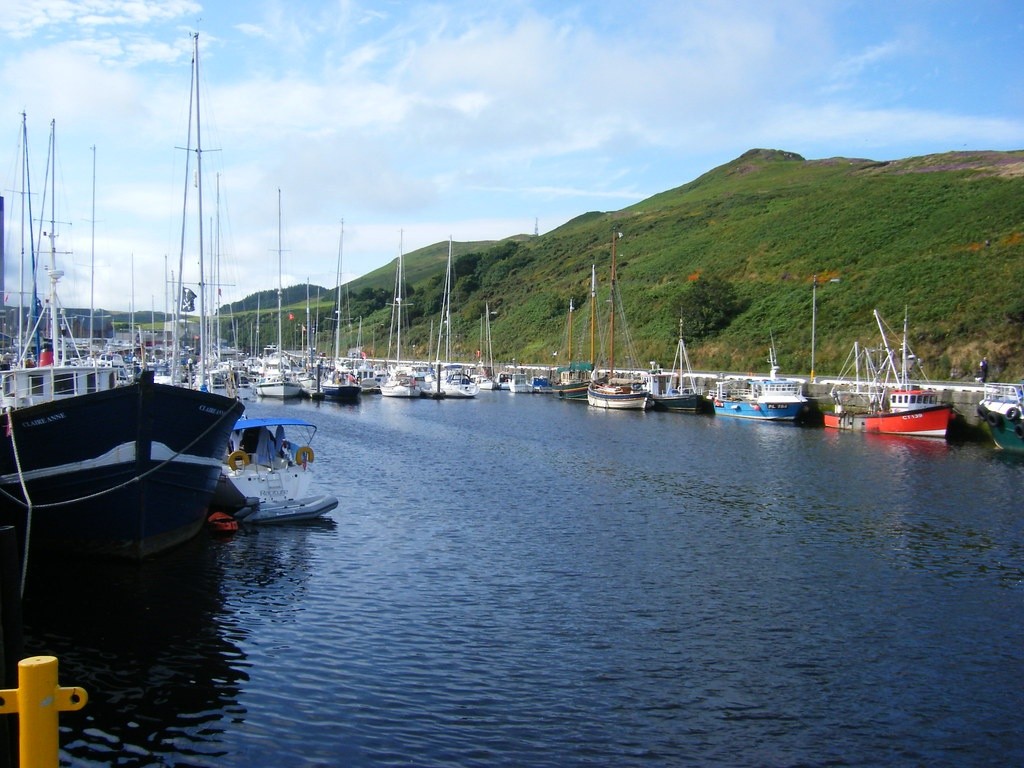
180, 288, 197, 312
290, 313, 294, 320
218, 288, 222, 295
3, 293, 9, 302
302, 326, 307, 331
195, 335, 199, 340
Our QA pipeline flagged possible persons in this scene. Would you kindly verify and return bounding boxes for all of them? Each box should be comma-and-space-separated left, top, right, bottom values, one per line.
978, 357, 990, 383
230, 427, 275, 455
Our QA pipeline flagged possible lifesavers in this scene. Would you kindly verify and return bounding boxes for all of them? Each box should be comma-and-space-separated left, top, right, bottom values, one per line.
296, 447, 314, 465
228, 450, 250, 471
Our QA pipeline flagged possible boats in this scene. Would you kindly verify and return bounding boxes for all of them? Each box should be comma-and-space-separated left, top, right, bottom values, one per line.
976, 376, 1024, 457
0, 368, 246, 613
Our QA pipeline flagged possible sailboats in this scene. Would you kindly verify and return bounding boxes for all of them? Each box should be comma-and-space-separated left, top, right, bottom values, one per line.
648, 306, 711, 413
192, 34, 342, 527
2, 113, 594, 408
820, 301, 956, 441
588, 228, 657, 413
712, 327, 809, 421
